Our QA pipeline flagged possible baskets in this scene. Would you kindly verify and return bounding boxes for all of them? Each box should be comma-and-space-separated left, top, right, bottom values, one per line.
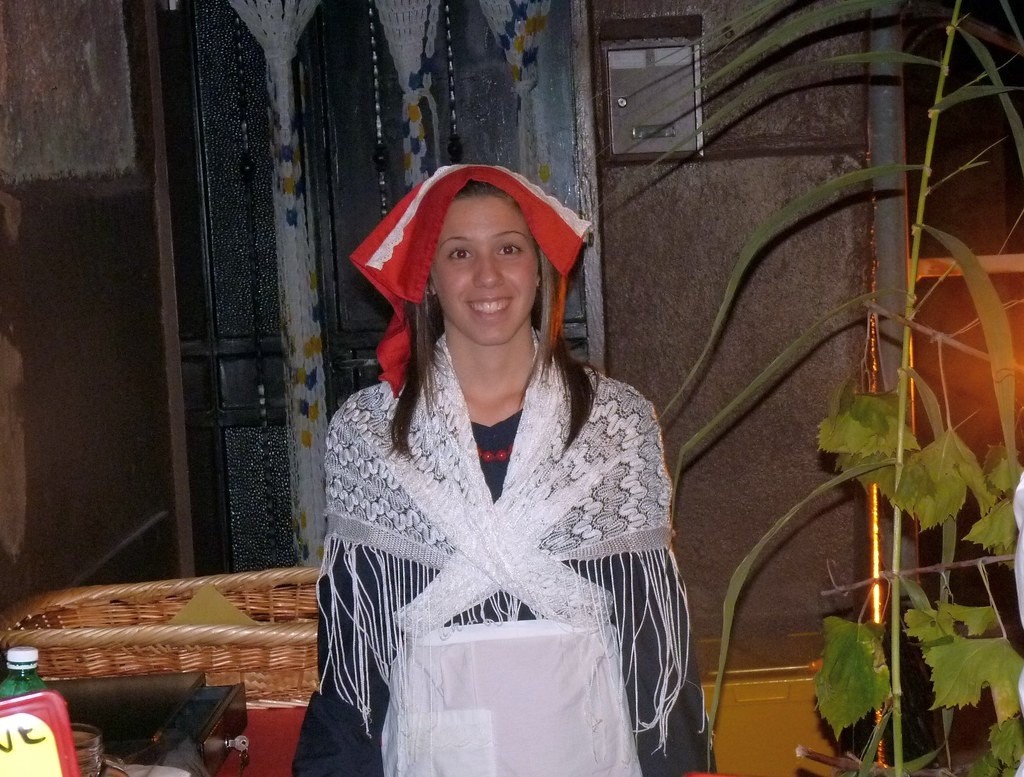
0, 568, 322, 704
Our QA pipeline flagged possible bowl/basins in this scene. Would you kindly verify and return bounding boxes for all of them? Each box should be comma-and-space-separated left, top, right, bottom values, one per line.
70, 723, 104, 777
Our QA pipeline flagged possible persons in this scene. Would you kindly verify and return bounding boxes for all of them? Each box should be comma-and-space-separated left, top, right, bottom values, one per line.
292, 165, 717, 777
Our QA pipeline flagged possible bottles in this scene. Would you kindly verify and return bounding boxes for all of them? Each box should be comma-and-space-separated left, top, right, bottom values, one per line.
0, 646, 48, 699
